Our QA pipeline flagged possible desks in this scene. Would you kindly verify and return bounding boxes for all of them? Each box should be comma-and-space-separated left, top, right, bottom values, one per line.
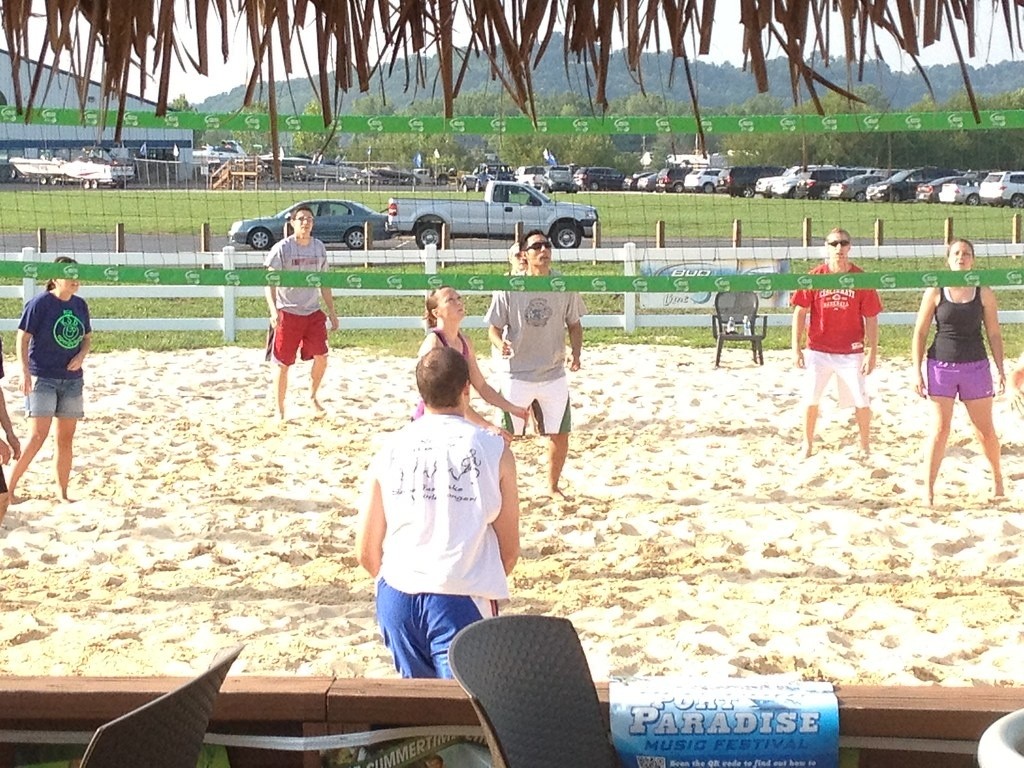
325, 677, 1024, 768
0, 674, 336, 768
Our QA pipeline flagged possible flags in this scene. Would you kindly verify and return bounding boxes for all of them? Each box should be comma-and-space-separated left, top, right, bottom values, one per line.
413, 149, 441, 168
136, 143, 179, 158
542, 150, 557, 168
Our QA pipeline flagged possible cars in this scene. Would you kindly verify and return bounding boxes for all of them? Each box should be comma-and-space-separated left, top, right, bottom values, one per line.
770, 164, 835, 199
682, 168, 722, 194
623, 170, 652, 192
636, 173, 659, 194
866, 169, 961, 203
459, 162, 582, 193
827, 174, 885, 203
227, 198, 396, 249
916, 177, 972, 204
655, 165, 691, 193
755, 166, 800, 198
795, 168, 869, 201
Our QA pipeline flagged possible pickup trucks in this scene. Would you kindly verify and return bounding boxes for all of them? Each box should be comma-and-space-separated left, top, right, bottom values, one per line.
940, 184, 983, 208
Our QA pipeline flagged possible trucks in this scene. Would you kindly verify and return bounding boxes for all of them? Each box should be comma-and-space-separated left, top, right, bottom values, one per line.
385, 180, 600, 248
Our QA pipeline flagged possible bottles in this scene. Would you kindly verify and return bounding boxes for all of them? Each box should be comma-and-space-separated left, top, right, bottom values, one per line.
743, 315, 752, 337
726, 317, 734, 333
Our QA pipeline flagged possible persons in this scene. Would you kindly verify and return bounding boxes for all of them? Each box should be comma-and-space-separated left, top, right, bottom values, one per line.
0, 257, 92, 522
413, 229, 585, 503
790, 227, 882, 464
912, 238, 1007, 507
265, 205, 340, 432
354, 348, 521, 681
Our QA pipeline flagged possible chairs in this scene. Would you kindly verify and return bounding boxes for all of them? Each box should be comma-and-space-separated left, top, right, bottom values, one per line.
711, 291, 768, 368
78, 643, 250, 768
446, 613, 617, 768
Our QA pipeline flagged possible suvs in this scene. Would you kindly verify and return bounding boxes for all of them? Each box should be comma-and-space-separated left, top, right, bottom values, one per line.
571, 167, 626, 191
962, 170, 996, 186
715, 164, 788, 199
978, 171, 1024, 209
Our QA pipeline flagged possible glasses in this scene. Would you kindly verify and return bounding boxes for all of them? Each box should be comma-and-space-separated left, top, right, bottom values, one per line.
294, 216, 315, 223
828, 240, 849, 247
525, 242, 551, 251
